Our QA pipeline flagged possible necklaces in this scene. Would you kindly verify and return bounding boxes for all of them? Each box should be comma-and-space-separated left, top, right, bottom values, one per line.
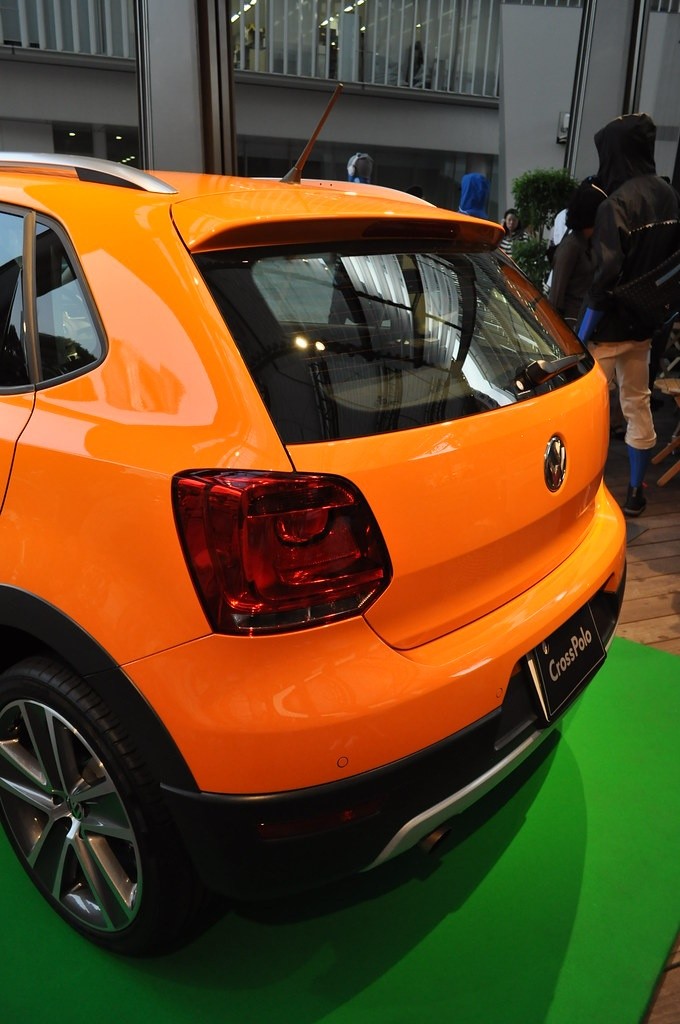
506, 234, 519, 242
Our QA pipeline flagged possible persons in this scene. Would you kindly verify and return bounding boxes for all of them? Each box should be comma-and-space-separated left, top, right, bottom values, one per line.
346, 152, 373, 185
577, 112, 679, 515
458, 172, 494, 220
499, 208, 530, 261
550, 176, 612, 336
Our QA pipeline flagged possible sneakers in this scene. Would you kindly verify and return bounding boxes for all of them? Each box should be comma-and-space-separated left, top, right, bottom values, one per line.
624, 486, 647, 516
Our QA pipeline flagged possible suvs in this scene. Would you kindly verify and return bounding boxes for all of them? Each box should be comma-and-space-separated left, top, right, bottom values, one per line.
0, 147, 629, 962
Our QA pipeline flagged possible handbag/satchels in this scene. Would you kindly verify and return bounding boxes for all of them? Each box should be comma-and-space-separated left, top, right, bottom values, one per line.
578, 220, 678, 342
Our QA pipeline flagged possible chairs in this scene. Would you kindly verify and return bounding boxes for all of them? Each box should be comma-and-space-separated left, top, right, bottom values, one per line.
291, 275, 409, 373
